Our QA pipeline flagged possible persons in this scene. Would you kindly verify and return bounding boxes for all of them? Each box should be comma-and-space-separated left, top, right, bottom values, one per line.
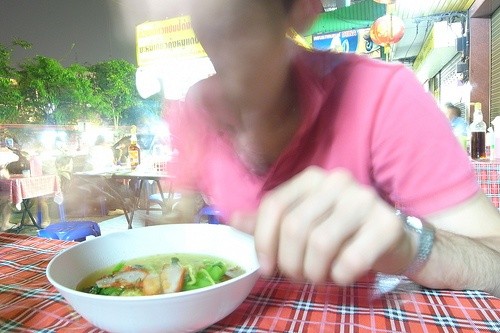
167, 0, 500, 299
444, 106, 470, 149
0, 134, 174, 233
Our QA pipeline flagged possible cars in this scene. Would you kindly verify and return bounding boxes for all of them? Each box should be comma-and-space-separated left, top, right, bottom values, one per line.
112, 135, 169, 159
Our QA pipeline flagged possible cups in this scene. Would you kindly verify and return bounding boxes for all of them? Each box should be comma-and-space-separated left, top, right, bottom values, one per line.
22, 170, 30, 178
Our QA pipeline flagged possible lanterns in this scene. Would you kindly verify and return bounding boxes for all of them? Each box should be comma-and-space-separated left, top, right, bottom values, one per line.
369, 14, 405, 52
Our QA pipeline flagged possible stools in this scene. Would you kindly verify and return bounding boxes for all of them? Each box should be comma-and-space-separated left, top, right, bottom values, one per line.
195, 206, 231, 226
37, 221, 102, 242
145, 192, 186, 227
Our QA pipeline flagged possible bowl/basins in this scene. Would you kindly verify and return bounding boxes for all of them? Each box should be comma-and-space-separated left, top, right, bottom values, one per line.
45, 222, 263, 332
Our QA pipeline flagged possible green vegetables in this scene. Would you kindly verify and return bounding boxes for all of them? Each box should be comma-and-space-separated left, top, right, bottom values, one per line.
82, 262, 226, 297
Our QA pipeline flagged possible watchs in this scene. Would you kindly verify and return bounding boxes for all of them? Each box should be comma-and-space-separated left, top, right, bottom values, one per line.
395, 209, 434, 279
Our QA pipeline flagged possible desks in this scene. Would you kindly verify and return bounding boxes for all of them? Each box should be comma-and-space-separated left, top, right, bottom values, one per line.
469, 155, 500, 215
0, 230, 500, 333
74, 166, 180, 229
0, 170, 67, 233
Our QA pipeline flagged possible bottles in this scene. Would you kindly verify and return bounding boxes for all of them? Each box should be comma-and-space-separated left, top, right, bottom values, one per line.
129, 124, 140, 170
471, 103, 486, 159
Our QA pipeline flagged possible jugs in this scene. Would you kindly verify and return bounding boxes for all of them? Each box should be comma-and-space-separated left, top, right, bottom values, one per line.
29, 156, 42, 177
